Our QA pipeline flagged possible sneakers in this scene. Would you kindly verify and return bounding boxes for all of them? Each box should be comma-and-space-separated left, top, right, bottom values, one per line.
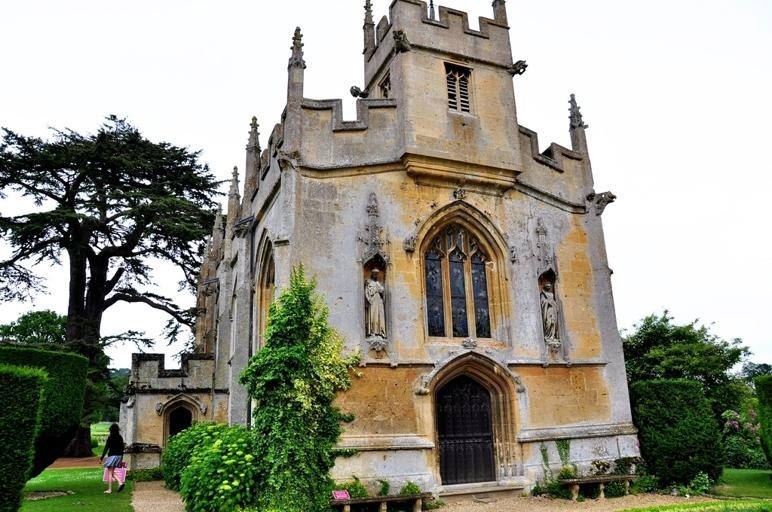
104, 490, 112, 494
117, 484, 125, 492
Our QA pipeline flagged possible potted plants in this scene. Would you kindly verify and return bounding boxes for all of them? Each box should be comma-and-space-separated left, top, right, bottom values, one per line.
615, 456, 643, 475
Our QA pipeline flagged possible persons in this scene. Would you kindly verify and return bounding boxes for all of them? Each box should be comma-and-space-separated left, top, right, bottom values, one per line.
539, 282, 559, 345
99, 422, 126, 494
365, 267, 387, 338
